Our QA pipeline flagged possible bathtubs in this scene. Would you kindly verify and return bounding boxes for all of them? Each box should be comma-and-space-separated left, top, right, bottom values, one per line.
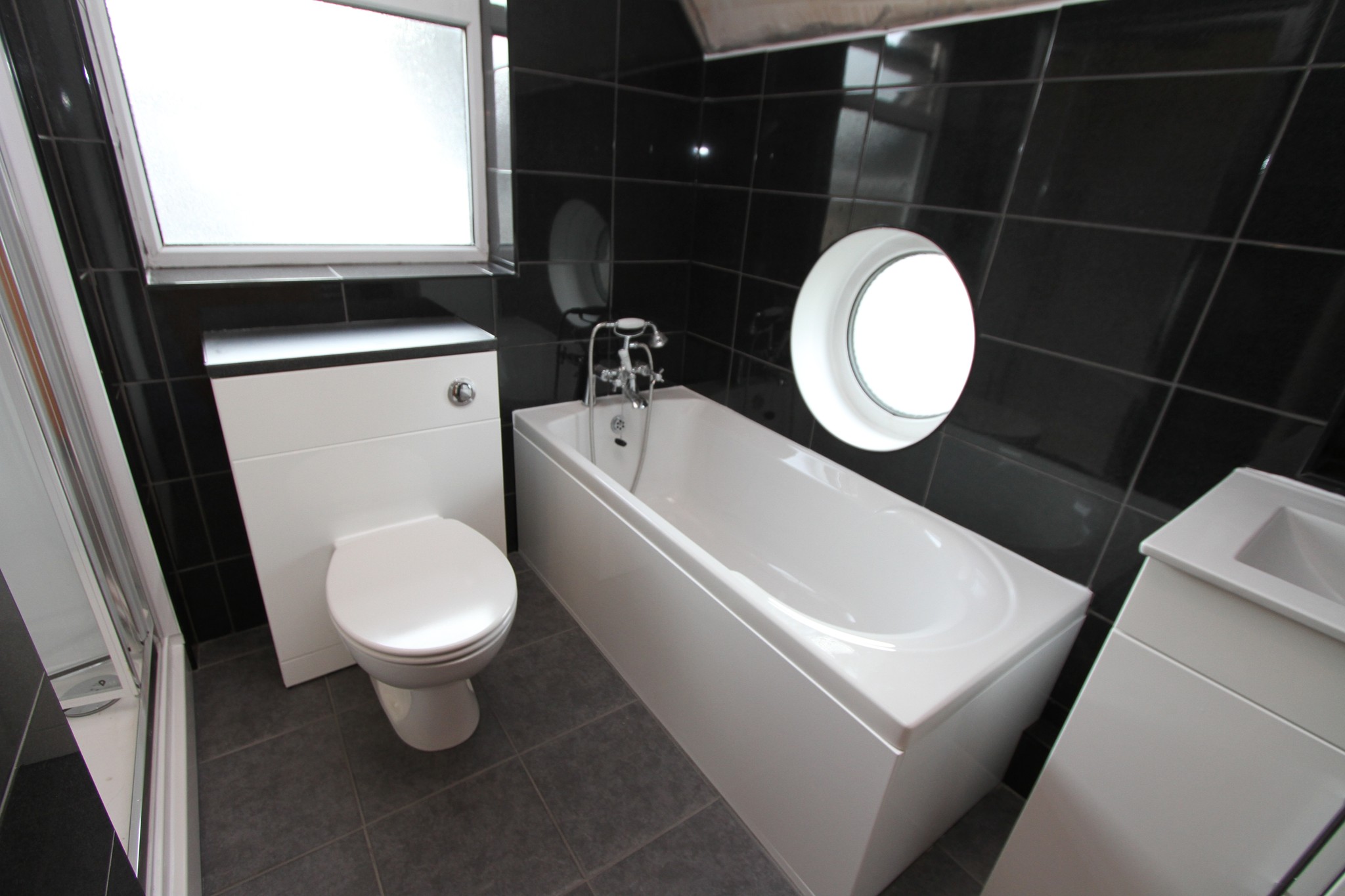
512, 384, 1098, 895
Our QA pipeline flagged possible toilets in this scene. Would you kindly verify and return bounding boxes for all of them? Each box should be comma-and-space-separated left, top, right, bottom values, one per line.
328, 515, 517, 754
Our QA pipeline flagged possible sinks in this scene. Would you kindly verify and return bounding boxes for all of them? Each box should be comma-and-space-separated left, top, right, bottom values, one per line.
1232, 502, 1344, 607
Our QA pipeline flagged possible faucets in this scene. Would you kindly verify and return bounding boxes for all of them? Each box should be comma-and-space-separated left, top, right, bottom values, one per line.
583, 365, 648, 410
